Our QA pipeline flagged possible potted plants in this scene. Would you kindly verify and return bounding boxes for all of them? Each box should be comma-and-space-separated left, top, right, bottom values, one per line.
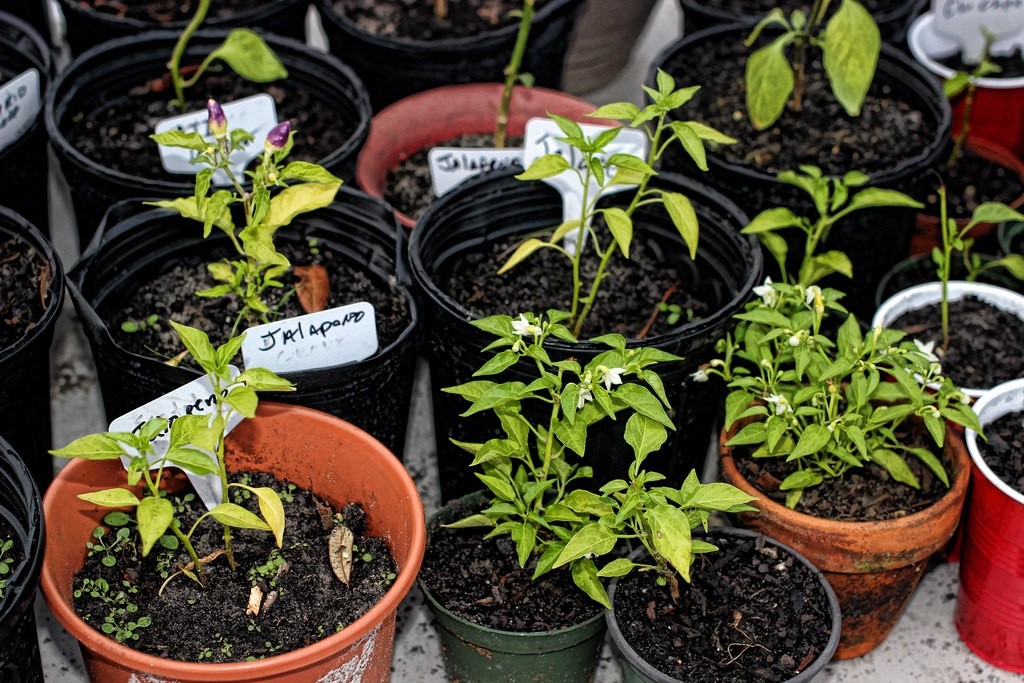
0, 0, 1024, 683
